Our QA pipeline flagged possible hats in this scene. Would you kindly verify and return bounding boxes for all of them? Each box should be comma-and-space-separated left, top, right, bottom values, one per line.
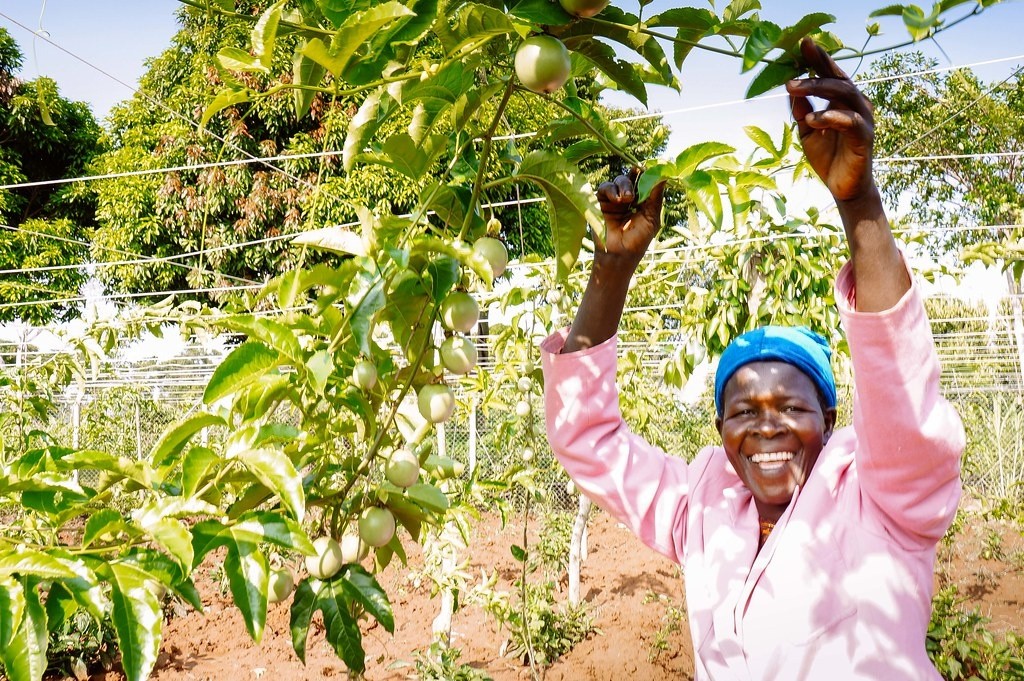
715, 325, 837, 416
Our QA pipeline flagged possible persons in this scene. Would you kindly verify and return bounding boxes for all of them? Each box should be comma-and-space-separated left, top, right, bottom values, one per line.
538, 38, 965, 681
